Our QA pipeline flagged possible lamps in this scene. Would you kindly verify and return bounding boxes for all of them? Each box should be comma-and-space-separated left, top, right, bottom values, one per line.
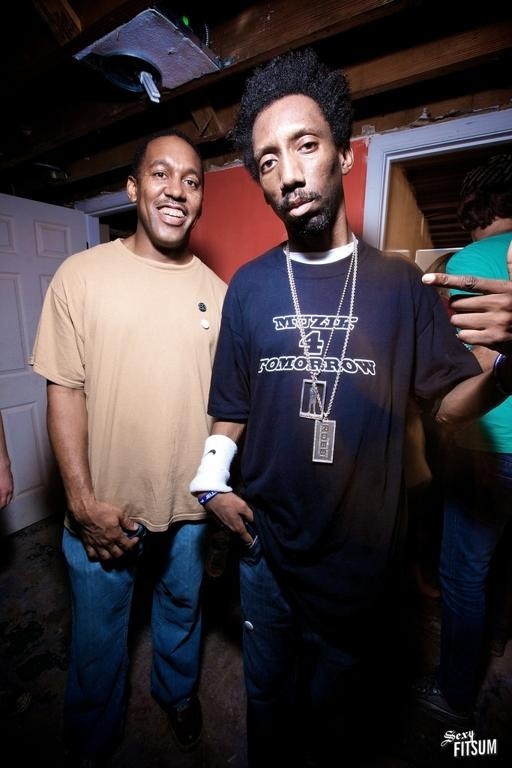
135, 69, 162, 104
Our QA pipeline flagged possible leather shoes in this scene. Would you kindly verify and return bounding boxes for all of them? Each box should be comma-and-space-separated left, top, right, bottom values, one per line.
167, 695, 204, 748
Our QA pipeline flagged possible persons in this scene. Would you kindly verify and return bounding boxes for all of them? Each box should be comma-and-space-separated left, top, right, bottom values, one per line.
19, 127, 236, 768
0, 409, 16, 520
398, 147, 511, 724
182, 50, 510, 767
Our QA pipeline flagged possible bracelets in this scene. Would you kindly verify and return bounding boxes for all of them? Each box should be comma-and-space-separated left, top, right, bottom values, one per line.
198, 491, 220, 505
492, 354, 511, 400
186, 432, 239, 498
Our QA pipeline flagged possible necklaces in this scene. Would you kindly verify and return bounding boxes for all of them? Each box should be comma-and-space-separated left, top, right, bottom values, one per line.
275, 237, 359, 465
